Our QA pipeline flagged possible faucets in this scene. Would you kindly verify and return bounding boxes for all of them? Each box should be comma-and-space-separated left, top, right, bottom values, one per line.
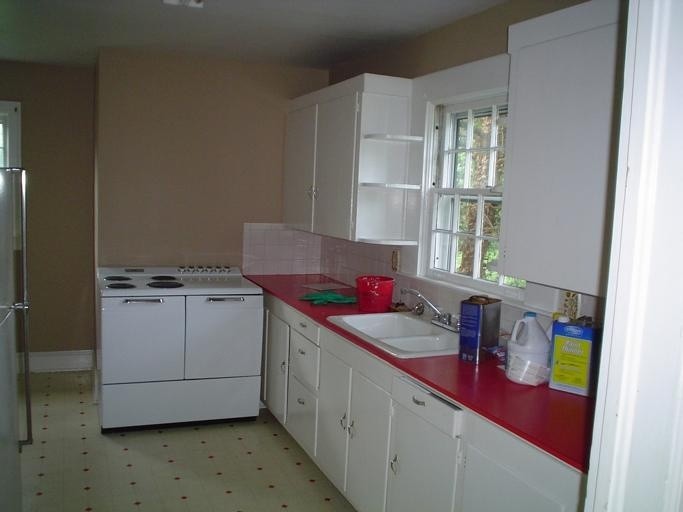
400, 287, 442, 317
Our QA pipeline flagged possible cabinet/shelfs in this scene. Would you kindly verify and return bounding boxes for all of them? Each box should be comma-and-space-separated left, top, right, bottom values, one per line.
358, 133, 424, 246
263, 295, 588, 512
282, 73, 413, 242
497, 0, 626, 297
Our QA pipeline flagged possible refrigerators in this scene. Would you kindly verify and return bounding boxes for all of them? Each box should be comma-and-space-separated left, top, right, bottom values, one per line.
0, 165, 33, 511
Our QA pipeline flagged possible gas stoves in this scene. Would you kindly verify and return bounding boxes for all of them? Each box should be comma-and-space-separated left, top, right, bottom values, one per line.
94, 266, 184, 293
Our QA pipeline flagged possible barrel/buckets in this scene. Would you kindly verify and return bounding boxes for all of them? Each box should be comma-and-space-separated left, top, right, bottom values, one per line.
548, 315, 603, 399
355, 275, 395, 314
545, 316, 570, 342
505, 312, 552, 387
457, 294, 502, 365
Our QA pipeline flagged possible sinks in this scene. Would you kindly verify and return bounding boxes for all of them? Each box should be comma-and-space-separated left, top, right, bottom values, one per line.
371, 335, 462, 360
325, 311, 445, 340
